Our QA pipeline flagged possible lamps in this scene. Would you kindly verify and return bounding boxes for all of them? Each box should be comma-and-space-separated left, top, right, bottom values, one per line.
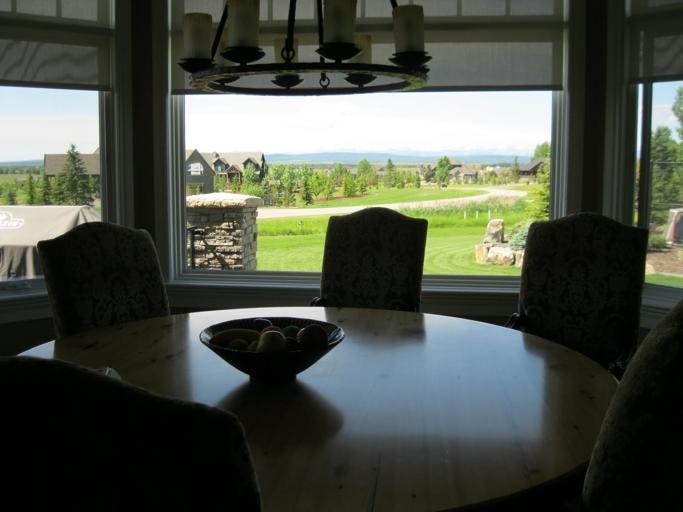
178, 0, 430, 94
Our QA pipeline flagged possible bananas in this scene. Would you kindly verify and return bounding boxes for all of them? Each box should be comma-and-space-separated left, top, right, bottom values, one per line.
210, 329, 260, 344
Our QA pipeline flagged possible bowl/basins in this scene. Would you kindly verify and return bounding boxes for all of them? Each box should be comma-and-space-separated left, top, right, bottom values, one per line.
200, 317, 345, 379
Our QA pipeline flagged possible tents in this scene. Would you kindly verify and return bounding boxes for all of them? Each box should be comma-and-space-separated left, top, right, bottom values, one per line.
0, 203, 104, 280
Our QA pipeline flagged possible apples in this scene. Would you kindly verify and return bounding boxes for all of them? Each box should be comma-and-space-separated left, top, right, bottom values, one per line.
257, 331, 286, 352
